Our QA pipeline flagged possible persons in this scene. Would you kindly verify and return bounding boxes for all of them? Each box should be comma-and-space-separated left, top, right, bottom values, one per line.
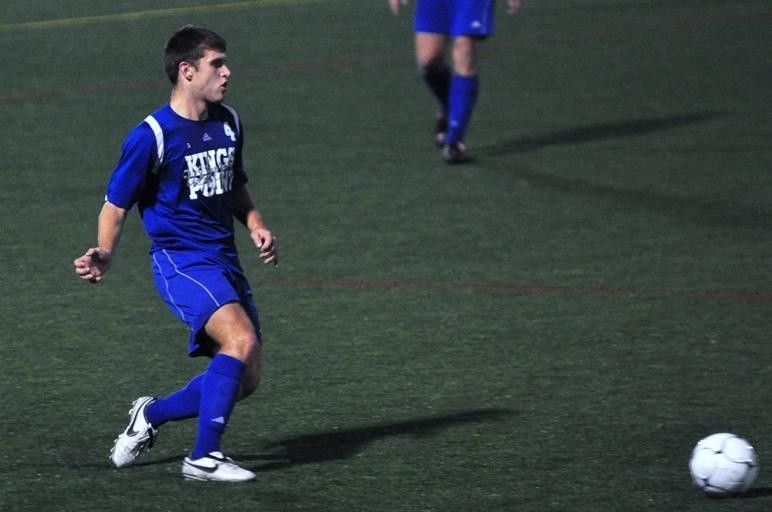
74, 26, 278, 483
388, 0, 521, 162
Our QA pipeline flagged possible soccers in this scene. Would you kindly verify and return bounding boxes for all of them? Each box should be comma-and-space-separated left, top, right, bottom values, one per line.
688, 432, 761, 500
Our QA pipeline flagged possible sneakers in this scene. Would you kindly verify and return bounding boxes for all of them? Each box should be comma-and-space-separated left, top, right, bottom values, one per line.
181, 451, 256, 483
434, 108, 467, 164
109, 396, 159, 468
